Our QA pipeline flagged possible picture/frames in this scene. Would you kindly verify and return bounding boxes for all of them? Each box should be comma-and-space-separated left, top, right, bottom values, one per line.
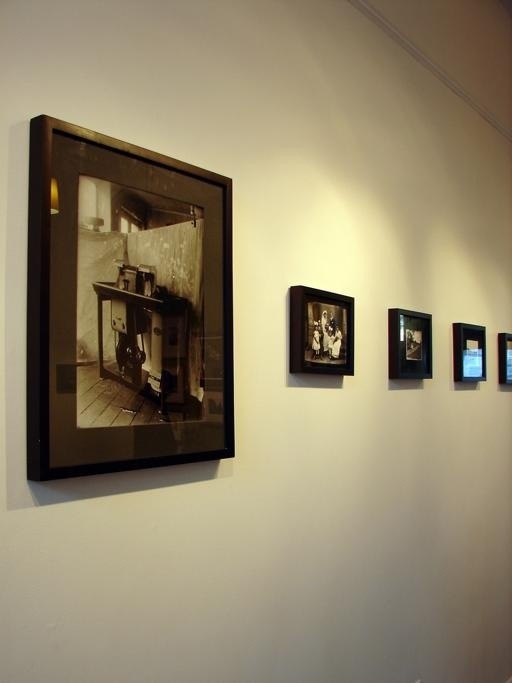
388, 308, 433, 379
27, 115, 234, 482
289, 286, 355, 375
453, 323, 511, 386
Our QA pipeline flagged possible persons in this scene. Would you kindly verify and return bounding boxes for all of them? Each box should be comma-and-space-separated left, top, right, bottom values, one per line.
311, 310, 343, 360
